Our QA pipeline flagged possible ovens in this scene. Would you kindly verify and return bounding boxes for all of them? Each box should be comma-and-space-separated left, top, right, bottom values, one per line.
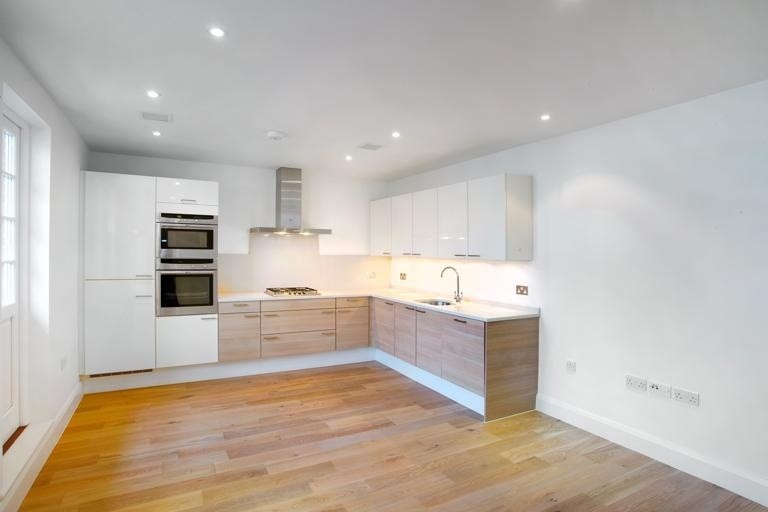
155, 212, 219, 317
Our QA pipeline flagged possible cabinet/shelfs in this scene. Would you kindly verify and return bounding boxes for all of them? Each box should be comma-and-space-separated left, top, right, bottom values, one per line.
217, 296, 370, 377
391, 188, 437, 258
371, 296, 394, 369
437, 173, 533, 262
442, 313, 540, 425
80, 170, 220, 396
370, 196, 392, 256
395, 303, 442, 393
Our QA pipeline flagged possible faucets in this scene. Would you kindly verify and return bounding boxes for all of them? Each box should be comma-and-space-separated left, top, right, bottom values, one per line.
441, 266, 463, 302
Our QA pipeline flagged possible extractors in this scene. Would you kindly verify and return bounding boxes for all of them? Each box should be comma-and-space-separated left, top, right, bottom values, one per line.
250, 167, 331, 238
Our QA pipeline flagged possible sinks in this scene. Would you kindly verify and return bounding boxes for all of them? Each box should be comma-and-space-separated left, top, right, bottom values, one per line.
415, 298, 455, 306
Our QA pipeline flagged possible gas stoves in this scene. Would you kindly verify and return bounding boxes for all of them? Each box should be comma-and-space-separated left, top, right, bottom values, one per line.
263, 286, 322, 298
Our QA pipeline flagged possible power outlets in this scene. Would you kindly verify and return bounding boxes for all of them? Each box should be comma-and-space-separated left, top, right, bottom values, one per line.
625, 374, 700, 407
